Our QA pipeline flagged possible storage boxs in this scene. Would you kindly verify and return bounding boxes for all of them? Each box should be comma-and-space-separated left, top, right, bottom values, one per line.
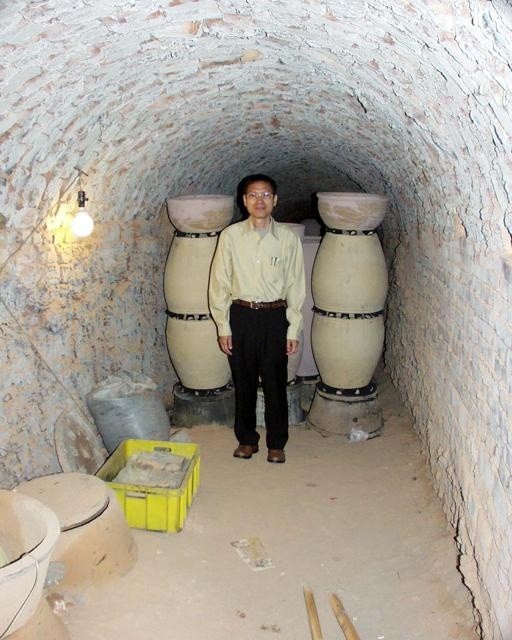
95, 439, 201, 533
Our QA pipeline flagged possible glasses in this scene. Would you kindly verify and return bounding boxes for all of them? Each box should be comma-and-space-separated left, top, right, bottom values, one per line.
246, 192, 273, 201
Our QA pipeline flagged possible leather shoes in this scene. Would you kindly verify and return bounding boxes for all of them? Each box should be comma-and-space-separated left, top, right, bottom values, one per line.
267, 448, 285, 463
233, 445, 259, 458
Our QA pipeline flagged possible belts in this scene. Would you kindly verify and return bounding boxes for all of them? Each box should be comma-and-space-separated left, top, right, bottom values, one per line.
232, 299, 286, 310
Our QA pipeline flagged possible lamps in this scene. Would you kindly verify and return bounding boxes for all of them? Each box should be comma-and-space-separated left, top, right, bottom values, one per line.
70, 190, 93, 238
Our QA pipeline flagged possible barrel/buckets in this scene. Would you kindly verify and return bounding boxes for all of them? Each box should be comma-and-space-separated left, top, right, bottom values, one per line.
0, 488, 63, 639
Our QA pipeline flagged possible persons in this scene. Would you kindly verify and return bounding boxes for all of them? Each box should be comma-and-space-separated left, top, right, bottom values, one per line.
206, 172, 307, 466
